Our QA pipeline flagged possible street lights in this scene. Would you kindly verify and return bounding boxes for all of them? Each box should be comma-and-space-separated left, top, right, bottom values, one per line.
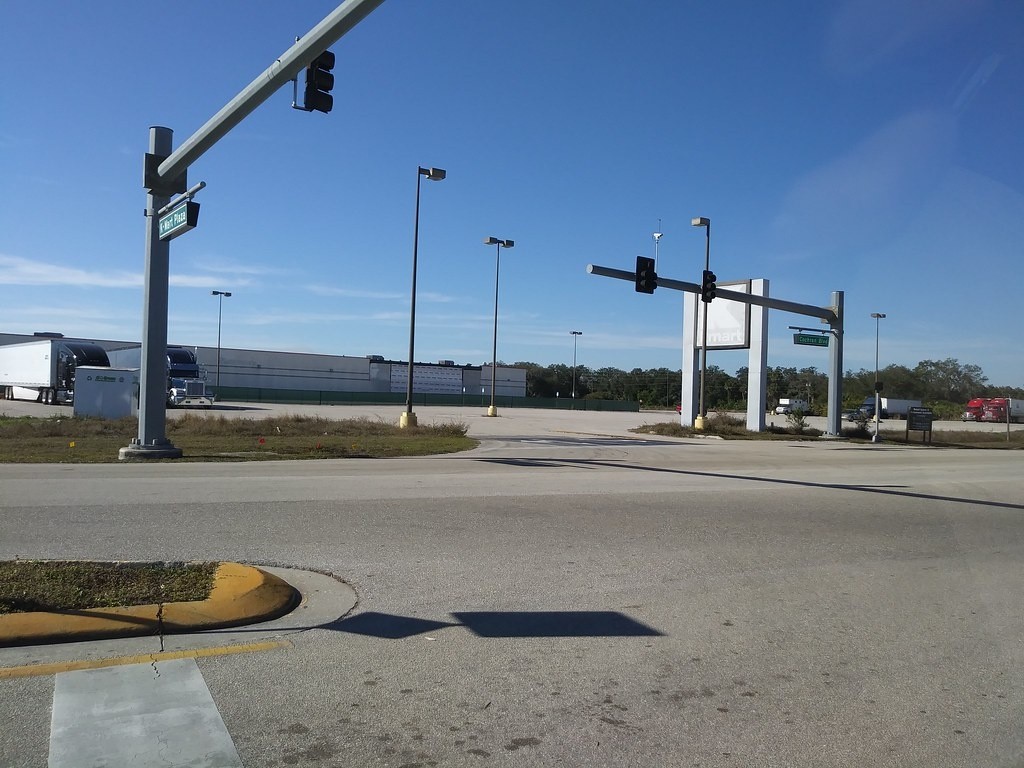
689, 215, 712, 432
398, 165, 446, 429
871, 312, 887, 443
482, 236, 516, 416
569, 330, 582, 411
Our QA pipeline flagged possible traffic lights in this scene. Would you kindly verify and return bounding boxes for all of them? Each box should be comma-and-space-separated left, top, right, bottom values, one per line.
303, 50, 335, 115
635, 256, 658, 295
211, 290, 232, 401
701, 270, 717, 303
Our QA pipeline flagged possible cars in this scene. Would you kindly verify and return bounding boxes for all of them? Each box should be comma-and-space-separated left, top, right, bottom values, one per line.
840, 408, 866, 424
676, 406, 708, 418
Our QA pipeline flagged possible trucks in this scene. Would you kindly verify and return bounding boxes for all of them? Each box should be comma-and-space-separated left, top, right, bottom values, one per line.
961, 398, 995, 423
983, 398, 1024, 424
0, 339, 112, 406
776, 398, 811, 415
860, 397, 923, 423
105, 345, 217, 411
